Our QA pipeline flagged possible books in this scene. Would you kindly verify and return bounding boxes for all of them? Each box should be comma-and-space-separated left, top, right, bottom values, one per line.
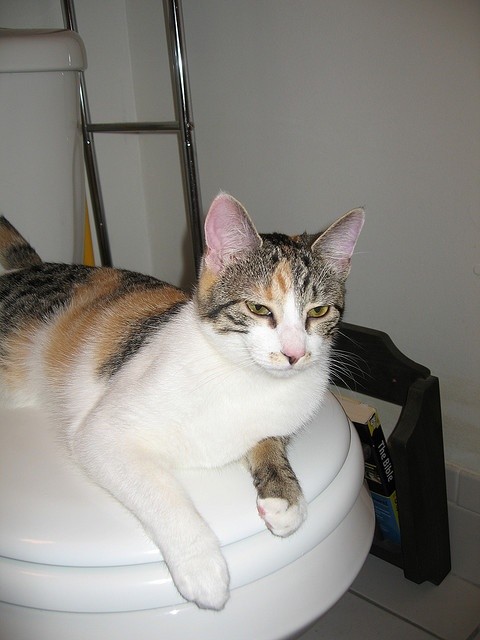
318, 395, 411, 551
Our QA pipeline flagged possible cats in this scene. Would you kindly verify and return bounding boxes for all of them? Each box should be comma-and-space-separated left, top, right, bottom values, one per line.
0, 191, 366, 612
